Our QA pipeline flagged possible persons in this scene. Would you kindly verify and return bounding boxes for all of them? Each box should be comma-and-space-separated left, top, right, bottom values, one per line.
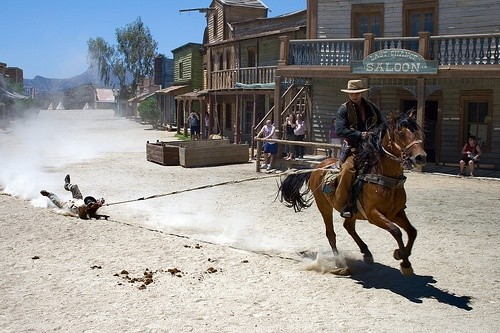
187, 109, 212, 140
253, 119, 276, 170
334, 80, 389, 218
282, 113, 307, 161
329, 117, 342, 159
457, 134, 481, 177
40, 174, 104, 220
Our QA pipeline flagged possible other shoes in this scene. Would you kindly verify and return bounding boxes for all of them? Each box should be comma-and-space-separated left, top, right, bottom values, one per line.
266, 164, 272, 170
284, 156, 292, 160
41, 191, 49, 196
65, 174, 70, 188
340, 209, 351, 218
259, 163, 268, 169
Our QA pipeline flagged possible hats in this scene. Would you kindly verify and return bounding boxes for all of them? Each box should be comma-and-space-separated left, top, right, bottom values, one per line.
340, 80, 371, 93
84, 196, 97, 205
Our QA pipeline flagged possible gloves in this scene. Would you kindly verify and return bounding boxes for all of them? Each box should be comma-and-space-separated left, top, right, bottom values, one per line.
361, 131, 374, 143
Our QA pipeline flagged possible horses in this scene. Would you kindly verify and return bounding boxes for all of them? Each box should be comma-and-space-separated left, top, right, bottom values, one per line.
272, 107, 426, 275
